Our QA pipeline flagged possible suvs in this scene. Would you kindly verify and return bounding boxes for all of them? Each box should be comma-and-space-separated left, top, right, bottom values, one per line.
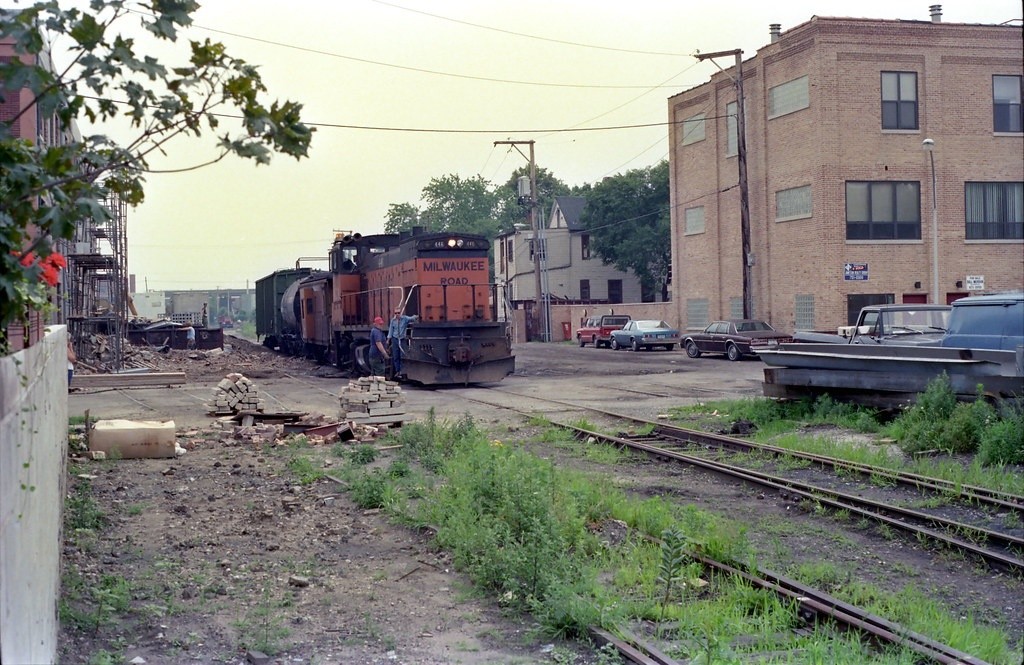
576, 314, 632, 348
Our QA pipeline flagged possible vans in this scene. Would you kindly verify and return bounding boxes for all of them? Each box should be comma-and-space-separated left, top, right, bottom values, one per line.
941, 293, 1024, 352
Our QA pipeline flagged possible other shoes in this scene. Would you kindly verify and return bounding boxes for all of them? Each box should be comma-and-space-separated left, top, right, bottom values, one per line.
394, 372, 400, 377
398, 375, 403, 379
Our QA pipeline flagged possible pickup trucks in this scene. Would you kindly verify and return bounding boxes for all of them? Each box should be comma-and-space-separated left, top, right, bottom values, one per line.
792, 303, 954, 346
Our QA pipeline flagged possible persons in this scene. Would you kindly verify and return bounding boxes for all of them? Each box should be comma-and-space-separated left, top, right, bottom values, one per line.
369, 316, 391, 379
176, 321, 196, 350
387, 307, 418, 377
67, 332, 75, 387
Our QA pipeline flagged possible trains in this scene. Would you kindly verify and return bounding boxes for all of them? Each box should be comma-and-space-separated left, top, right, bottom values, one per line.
254, 224, 517, 390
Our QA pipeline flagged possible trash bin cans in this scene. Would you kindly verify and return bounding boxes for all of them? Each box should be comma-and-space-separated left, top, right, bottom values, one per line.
195, 327, 224, 351
562, 321, 572, 341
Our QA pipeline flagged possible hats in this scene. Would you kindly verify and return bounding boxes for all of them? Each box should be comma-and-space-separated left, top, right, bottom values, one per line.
373, 316, 384, 325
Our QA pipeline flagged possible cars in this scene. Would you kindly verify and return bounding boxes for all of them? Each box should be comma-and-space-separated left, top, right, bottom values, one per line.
609, 319, 681, 352
679, 318, 793, 361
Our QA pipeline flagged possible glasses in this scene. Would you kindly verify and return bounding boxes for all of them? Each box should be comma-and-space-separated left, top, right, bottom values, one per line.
394, 312, 401, 315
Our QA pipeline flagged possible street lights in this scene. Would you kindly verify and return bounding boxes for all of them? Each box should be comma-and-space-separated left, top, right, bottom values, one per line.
920, 137, 943, 306
